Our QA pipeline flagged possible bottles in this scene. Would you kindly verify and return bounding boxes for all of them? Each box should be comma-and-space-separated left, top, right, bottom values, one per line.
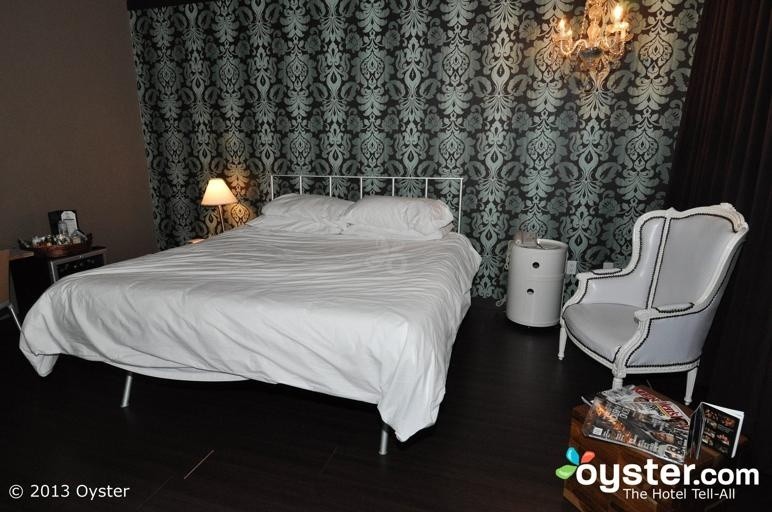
72, 232, 81, 245
57, 219, 69, 236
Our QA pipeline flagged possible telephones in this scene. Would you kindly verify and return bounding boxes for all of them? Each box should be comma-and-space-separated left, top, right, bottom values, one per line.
514, 230, 538, 248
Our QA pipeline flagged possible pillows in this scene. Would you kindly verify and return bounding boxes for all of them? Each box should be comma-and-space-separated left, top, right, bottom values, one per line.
243, 190, 455, 242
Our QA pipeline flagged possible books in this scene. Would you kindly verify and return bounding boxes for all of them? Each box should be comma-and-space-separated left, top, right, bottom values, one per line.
686, 402, 745, 459
581, 384, 691, 465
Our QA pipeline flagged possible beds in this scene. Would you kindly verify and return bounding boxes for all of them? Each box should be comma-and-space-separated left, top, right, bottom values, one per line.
57, 174, 468, 455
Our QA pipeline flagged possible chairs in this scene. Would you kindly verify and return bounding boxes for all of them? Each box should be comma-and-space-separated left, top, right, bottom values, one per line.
554, 199, 751, 406
1, 250, 23, 332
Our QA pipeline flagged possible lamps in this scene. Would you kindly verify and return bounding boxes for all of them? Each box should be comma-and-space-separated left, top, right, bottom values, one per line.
556, 0, 632, 75
200, 177, 238, 233
